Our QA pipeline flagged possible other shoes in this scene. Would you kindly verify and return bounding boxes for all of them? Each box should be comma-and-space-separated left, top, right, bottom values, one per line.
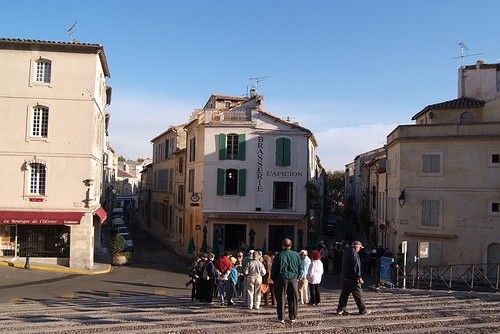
337, 309, 349, 315
278, 319, 284, 323
360, 309, 372, 314
287, 317, 296, 322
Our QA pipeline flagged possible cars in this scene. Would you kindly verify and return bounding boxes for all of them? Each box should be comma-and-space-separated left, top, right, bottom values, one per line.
110, 208, 129, 236
113, 233, 136, 254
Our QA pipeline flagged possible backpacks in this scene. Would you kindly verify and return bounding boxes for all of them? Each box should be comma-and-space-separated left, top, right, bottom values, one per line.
203, 261, 212, 280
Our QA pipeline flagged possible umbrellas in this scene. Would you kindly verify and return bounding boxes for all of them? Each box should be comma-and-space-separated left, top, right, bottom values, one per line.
187, 223, 219, 260
249, 228, 256, 248
297, 227, 317, 251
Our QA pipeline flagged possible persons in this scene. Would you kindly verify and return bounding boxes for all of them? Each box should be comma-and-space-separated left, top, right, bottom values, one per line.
190, 238, 393, 324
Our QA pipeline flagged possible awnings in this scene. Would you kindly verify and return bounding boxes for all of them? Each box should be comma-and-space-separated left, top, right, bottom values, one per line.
96, 208, 107, 224
0, 210, 85, 226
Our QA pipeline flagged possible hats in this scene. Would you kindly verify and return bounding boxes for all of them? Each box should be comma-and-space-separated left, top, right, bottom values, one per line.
249, 250, 255, 254
230, 257, 237, 263
352, 240, 364, 248
298, 250, 308, 256
